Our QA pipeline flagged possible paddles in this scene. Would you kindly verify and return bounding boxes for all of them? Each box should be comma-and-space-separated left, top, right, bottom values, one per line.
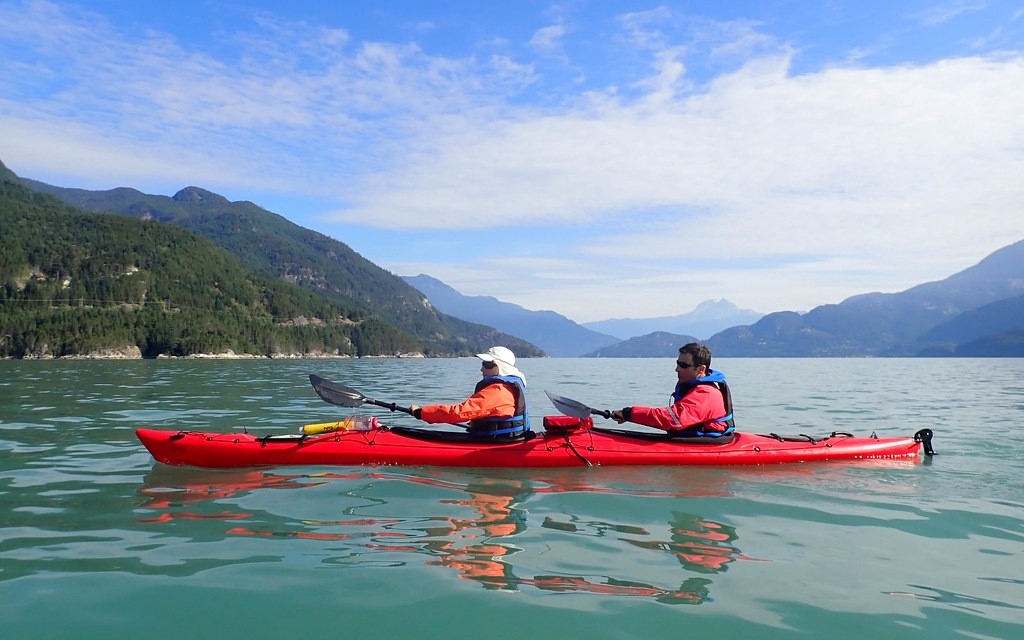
308, 372, 468, 428
543, 389, 667, 432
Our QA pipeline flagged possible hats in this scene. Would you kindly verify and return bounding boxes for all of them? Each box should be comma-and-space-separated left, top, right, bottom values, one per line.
476, 346, 527, 387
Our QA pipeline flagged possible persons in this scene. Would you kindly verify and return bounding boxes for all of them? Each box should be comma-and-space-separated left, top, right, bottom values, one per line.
612, 342, 736, 446
408, 346, 530, 442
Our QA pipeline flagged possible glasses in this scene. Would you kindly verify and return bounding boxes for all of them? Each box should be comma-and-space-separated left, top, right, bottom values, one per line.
482, 361, 494, 368
677, 360, 698, 368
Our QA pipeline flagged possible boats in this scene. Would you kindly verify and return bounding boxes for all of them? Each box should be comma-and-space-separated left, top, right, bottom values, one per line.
134, 413, 940, 468
